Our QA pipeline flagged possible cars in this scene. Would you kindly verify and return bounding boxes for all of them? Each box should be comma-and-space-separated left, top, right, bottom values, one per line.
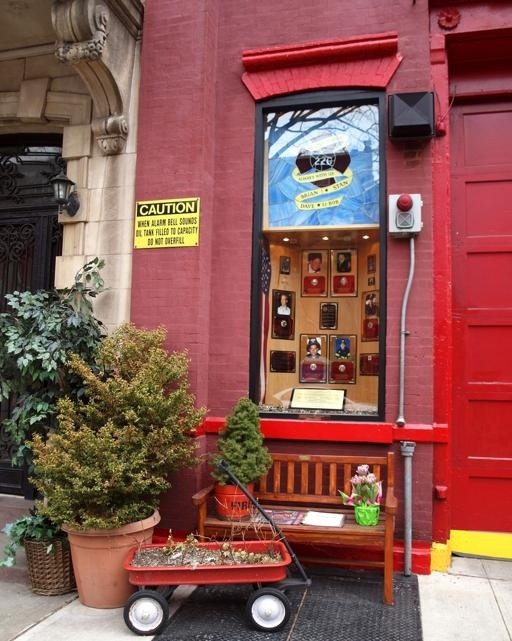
273, 387, 378, 415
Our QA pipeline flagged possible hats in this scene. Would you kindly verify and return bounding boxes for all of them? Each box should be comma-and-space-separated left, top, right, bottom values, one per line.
306, 337, 321, 351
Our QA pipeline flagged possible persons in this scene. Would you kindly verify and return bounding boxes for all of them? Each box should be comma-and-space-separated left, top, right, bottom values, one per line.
334, 338, 351, 363
307, 253, 322, 275
277, 293, 291, 317
364, 292, 377, 314
305, 336, 321, 360
336, 253, 351, 273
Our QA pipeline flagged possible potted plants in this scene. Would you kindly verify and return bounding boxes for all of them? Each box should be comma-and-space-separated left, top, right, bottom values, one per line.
207, 395, 273, 521
25, 320, 211, 610
0, 252, 109, 595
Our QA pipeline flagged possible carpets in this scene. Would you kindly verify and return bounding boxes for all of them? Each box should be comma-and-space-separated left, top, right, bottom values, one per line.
151, 567, 424, 641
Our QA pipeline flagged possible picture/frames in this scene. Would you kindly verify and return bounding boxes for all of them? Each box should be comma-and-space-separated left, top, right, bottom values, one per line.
270, 247, 380, 411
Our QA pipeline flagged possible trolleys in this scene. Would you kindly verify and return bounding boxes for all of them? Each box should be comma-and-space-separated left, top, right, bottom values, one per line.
120, 458, 313, 635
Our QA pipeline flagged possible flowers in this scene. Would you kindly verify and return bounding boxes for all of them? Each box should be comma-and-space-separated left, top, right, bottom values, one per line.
349, 464, 380, 507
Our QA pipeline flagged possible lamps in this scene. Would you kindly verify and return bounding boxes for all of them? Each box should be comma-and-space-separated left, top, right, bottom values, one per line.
50, 167, 80, 217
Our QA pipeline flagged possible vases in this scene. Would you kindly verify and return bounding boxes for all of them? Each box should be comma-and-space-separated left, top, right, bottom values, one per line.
354, 506, 380, 526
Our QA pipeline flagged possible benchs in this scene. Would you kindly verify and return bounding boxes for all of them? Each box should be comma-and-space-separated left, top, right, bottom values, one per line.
191, 449, 398, 604
